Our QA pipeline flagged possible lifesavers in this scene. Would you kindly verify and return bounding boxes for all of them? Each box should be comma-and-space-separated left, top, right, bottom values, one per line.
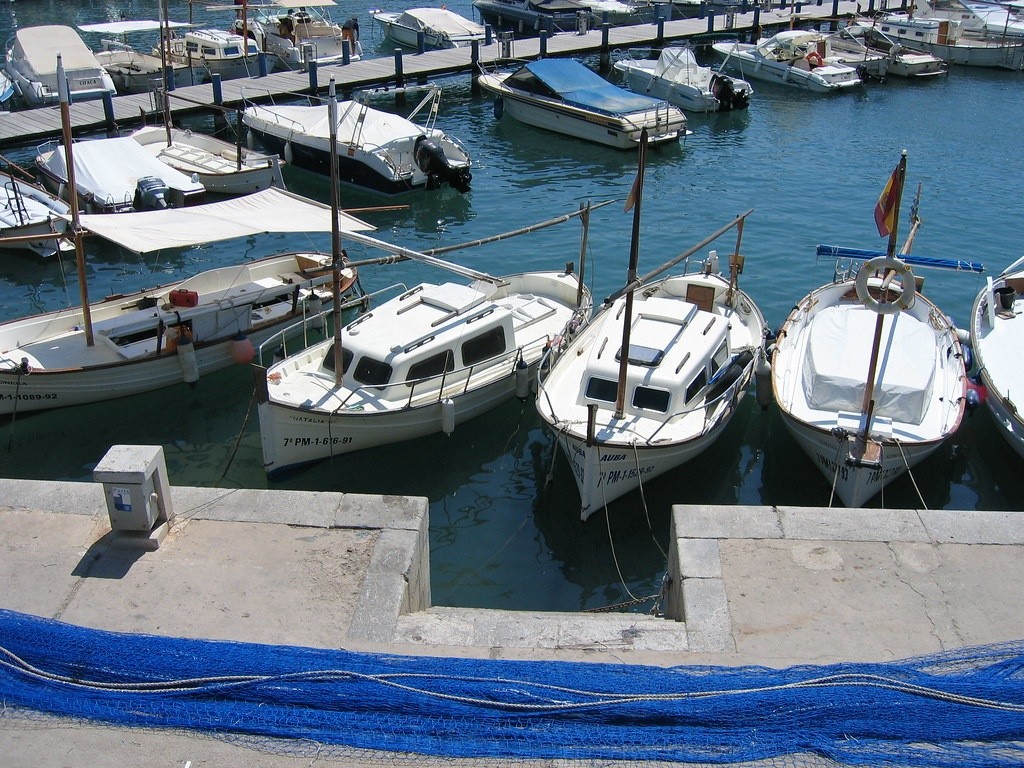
806, 51, 822, 67
855, 256, 916, 315
705, 350, 753, 405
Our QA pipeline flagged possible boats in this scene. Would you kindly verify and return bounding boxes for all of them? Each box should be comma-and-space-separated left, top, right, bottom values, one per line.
809, 22, 950, 79
756, 27, 889, 83
0, 66, 23, 103
534, 126, 770, 524
471, 0, 809, 34
75, 17, 190, 94
130, 106, 288, 196
476, 52, 695, 151
35, 136, 208, 215
612, 42, 755, 114
969, 254, 1024, 463
0, 170, 79, 258
875, 0, 1024, 46
714, 30, 866, 94
249, 70, 597, 478
4, 24, 117, 108
239, 73, 475, 191
847, 5, 1024, 72
228, 0, 364, 66
368, 1, 499, 53
770, 147, 972, 508
150, 0, 266, 80
0, 52, 360, 416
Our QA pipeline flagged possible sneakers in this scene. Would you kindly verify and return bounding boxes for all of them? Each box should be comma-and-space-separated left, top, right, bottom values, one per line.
352, 52, 357, 56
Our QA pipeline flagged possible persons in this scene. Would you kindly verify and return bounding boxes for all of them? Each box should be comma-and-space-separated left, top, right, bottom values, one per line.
282, 9, 293, 33
341, 18, 359, 56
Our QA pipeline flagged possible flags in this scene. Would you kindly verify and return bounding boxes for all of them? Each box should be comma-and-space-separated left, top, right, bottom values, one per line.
874, 169, 897, 238
